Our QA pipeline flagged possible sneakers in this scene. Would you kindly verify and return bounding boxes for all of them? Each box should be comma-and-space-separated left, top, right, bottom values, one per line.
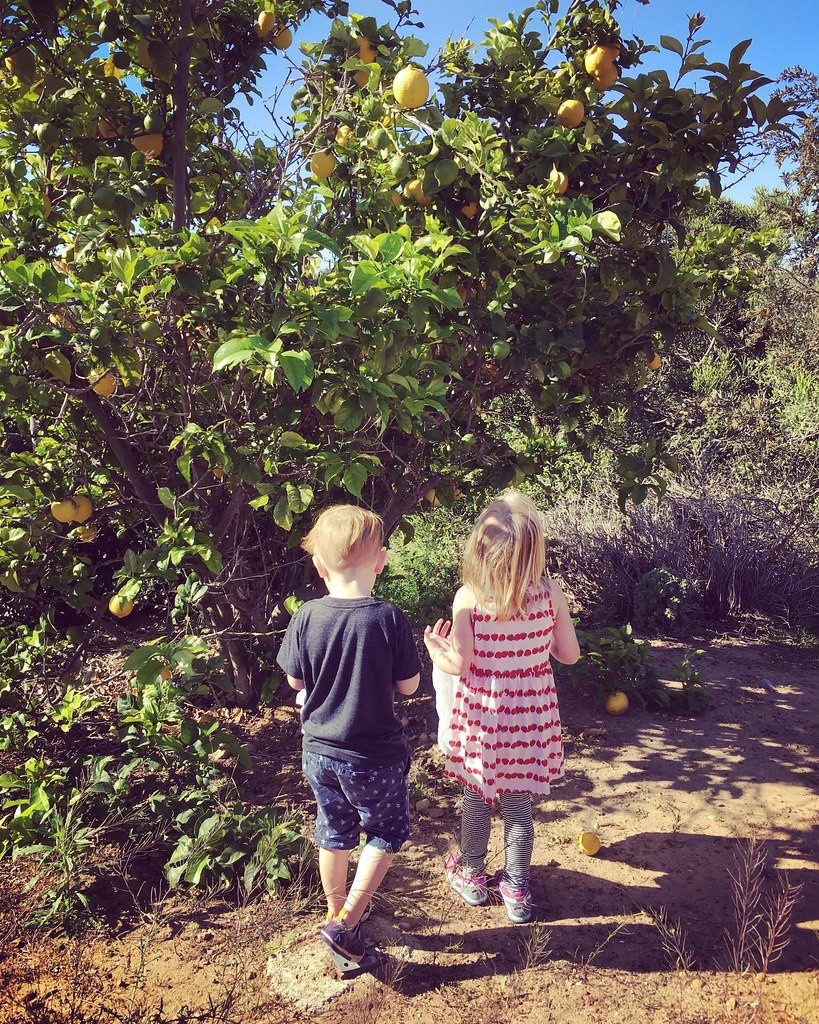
494, 869, 532, 922
320, 920, 366, 963
324, 900, 372, 927
444, 849, 488, 906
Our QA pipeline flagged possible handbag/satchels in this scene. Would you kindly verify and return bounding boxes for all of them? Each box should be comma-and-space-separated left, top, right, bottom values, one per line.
430, 633, 461, 755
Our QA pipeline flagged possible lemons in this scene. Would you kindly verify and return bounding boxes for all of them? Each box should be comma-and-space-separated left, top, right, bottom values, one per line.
646, 353, 660, 370
112, 778, 130, 797
5, 9, 621, 643
578, 832, 600, 855
605, 691, 629, 715
160, 667, 172, 680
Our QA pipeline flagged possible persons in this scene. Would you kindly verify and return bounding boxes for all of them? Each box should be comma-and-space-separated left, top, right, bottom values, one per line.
424, 493, 580, 925
274, 505, 422, 964
